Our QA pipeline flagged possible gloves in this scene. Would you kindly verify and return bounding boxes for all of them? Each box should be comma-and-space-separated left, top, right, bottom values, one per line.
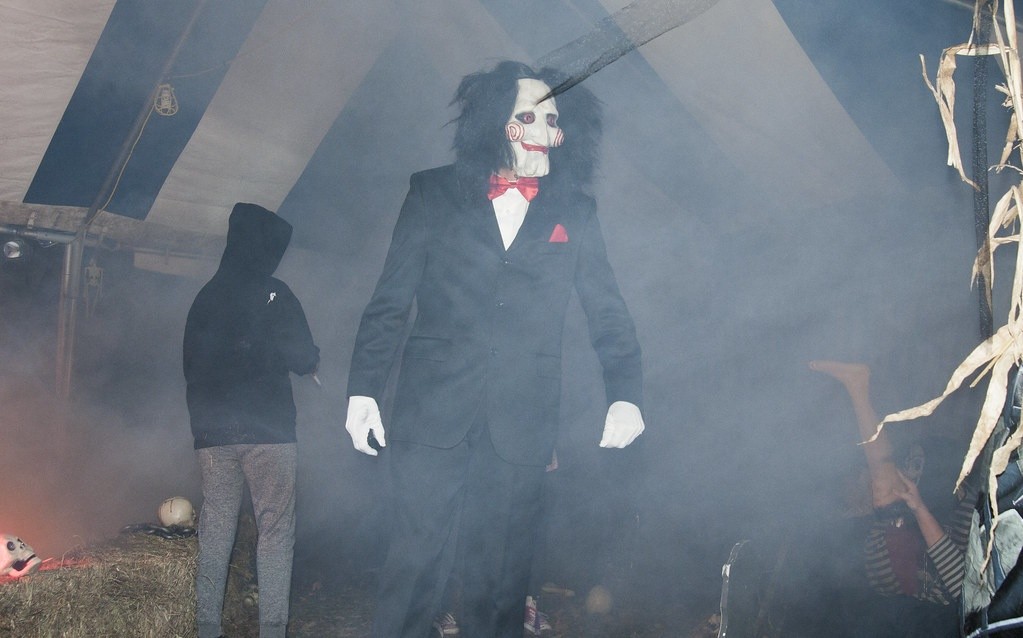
598, 401, 645, 449
345, 396, 386, 455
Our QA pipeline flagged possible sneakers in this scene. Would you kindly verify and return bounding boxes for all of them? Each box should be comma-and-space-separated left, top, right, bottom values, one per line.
430, 608, 461, 635
522, 595, 552, 634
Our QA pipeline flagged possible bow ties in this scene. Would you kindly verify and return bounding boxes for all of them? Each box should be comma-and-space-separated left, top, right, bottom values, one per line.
488, 174, 539, 202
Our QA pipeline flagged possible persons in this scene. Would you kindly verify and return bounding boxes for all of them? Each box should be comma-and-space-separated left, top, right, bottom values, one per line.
810, 359, 983, 638
183, 202, 321, 638
344, 61, 645, 638
